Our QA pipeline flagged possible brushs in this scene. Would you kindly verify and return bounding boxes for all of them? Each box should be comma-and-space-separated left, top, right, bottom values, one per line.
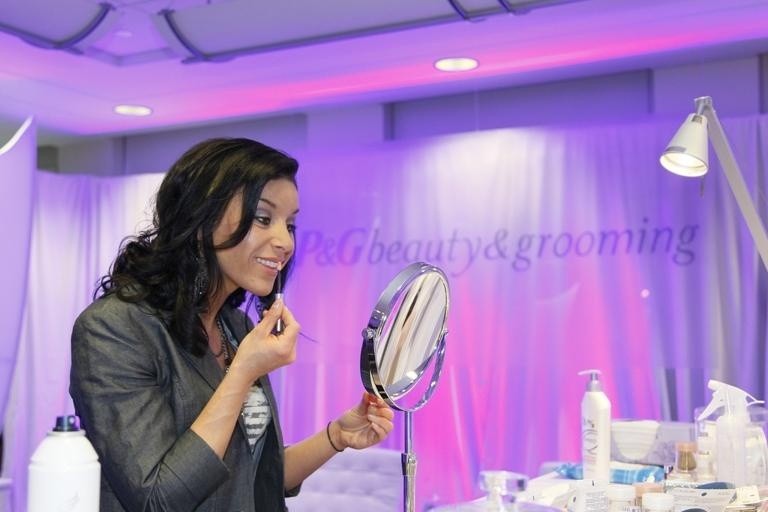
276, 261, 283, 331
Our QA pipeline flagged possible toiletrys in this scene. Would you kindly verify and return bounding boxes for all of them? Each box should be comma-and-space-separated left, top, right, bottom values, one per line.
578, 370, 612, 482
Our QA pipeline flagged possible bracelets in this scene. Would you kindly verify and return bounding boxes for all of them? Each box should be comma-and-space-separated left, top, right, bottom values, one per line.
326, 422, 345, 452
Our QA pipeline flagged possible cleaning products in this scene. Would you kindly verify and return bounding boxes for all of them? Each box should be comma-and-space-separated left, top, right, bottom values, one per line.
697, 378, 757, 488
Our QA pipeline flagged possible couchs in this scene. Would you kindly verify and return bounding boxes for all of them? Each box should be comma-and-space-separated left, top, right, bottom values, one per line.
283, 442, 404, 512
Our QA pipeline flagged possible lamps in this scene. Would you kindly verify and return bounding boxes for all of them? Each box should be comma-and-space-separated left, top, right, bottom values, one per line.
657, 94, 767, 276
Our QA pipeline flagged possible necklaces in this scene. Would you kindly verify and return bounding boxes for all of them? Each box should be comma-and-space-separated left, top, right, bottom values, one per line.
203, 316, 225, 358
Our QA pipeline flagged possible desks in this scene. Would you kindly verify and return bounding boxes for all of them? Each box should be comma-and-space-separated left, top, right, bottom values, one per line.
426, 456, 767, 512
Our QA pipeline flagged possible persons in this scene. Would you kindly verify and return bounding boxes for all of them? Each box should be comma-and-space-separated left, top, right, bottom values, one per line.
69, 140, 394, 512
